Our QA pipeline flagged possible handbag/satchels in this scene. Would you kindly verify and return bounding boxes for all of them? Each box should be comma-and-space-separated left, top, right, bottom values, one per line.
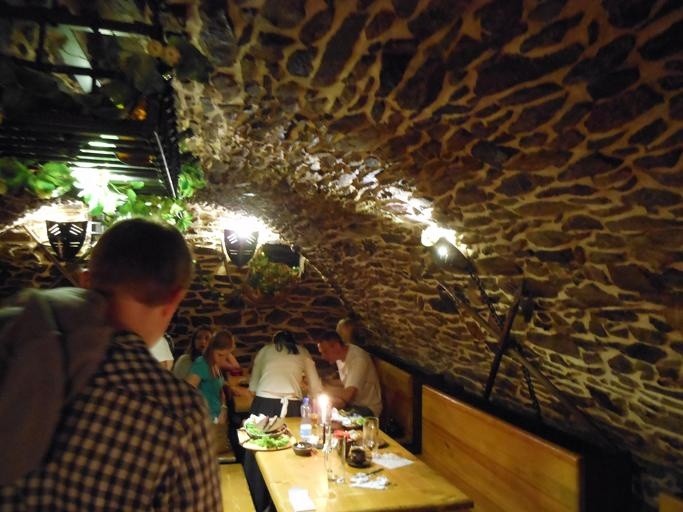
209, 416, 232, 456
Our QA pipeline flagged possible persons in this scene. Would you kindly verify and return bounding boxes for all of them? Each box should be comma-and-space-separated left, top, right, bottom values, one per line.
326, 318, 356, 379
173, 324, 240, 379
184, 329, 234, 454
244, 330, 323, 512
317, 331, 384, 420
0, 216, 223, 512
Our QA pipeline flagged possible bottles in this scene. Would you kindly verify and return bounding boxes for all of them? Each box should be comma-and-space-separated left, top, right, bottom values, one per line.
300, 397, 312, 440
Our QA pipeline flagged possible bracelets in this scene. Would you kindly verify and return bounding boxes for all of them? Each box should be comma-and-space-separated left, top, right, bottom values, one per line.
220, 404, 228, 409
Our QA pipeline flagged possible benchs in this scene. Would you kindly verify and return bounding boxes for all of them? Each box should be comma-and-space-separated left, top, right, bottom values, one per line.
369, 355, 418, 452
413, 383, 583, 511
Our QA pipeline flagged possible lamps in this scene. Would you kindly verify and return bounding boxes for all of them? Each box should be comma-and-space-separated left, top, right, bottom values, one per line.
418, 223, 545, 422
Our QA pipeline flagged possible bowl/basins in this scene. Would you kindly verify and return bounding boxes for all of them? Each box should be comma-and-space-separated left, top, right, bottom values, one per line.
243, 422, 287, 440
293, 442, 313, 456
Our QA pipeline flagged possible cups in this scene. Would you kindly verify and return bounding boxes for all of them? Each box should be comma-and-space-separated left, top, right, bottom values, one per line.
324, 434, 345, 481
362, 417, 379, 458
